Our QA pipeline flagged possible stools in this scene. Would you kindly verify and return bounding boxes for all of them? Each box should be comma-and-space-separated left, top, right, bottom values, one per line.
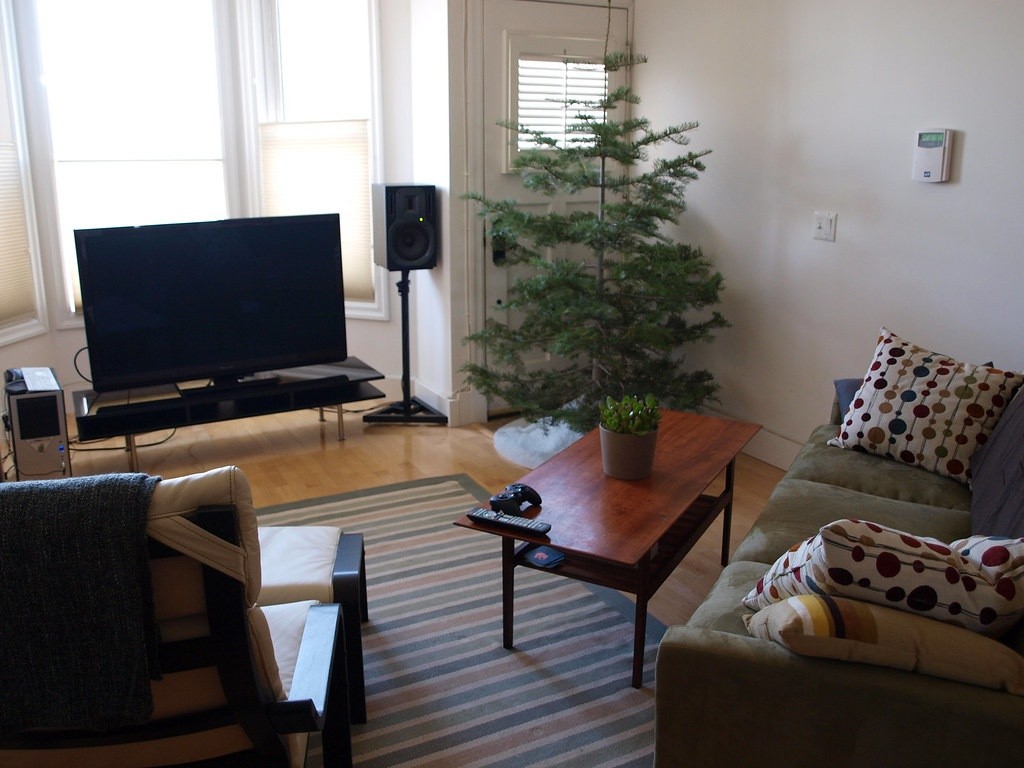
258, 526, 369, 726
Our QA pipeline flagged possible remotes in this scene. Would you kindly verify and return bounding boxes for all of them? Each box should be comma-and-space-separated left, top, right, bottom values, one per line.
466, 507, 551, 535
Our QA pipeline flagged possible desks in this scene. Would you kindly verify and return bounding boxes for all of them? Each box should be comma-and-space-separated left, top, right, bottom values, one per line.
71, 354, 387, 473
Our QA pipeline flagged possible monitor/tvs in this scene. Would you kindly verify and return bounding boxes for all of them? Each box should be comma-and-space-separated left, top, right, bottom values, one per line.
73, 213, 348, 398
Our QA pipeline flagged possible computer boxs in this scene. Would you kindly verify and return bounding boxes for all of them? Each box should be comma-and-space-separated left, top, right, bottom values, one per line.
4, 367, 72, 482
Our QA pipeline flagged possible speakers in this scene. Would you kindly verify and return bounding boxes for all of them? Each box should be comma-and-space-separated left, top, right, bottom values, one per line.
371, 183, 437, 271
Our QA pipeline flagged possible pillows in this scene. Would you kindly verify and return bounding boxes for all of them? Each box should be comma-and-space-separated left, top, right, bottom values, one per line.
825, 325, 1024, 491
833, 361, 994, 423
742, 593, 1024, 696
741, 517, 1024, 639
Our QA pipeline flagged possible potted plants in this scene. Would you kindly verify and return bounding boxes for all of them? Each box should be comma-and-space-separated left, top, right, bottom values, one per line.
597, 393, 664, 481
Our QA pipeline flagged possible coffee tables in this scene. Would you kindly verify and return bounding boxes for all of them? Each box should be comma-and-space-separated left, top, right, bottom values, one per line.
452, 410, 762, 690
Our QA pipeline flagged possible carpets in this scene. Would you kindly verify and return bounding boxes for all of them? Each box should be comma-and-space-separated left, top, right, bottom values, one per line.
254, 472, 668, 768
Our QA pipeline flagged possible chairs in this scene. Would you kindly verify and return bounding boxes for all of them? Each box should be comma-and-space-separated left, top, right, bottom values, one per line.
0, 464, 352, 768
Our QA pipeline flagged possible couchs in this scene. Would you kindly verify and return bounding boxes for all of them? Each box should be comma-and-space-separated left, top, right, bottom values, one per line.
653, 374, 1024, 768
